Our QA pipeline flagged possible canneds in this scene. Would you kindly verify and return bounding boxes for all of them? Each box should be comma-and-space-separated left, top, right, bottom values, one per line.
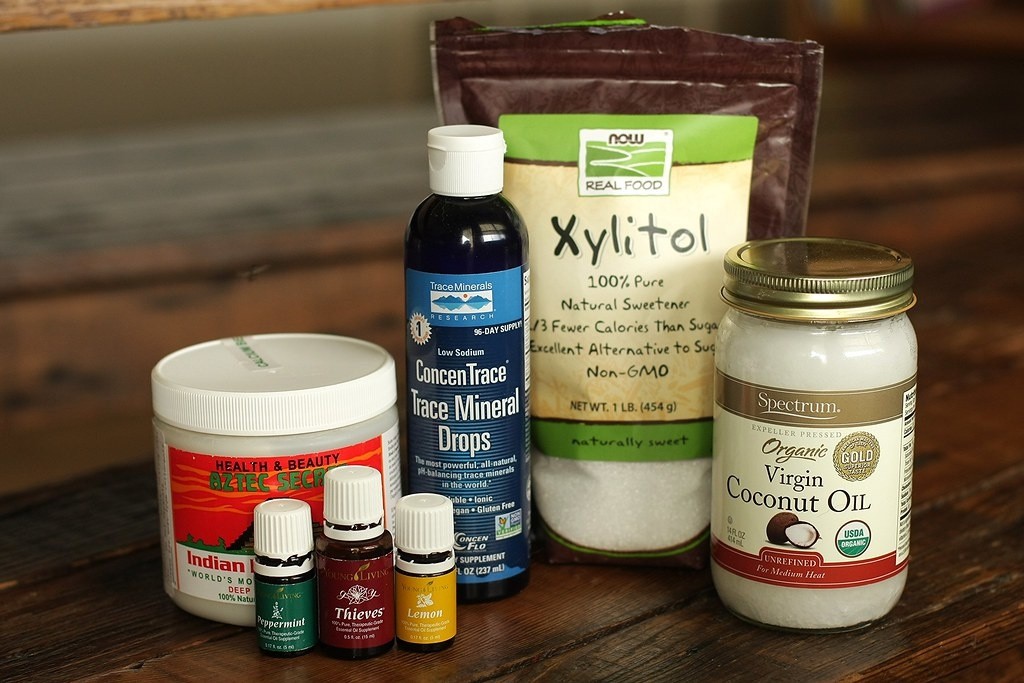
150, 332, 404, 630
709, 237, 918, 633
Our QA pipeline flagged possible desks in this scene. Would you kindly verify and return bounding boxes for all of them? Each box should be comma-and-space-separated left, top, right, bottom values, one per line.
0, 214, 1024, 683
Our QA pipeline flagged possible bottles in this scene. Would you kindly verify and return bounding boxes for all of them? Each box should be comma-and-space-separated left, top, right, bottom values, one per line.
315, 463, 394, 659
394, 492, 456, 652
403, 124, 534, 600
254, 498, 315, 658
712, 238, 918, 636
